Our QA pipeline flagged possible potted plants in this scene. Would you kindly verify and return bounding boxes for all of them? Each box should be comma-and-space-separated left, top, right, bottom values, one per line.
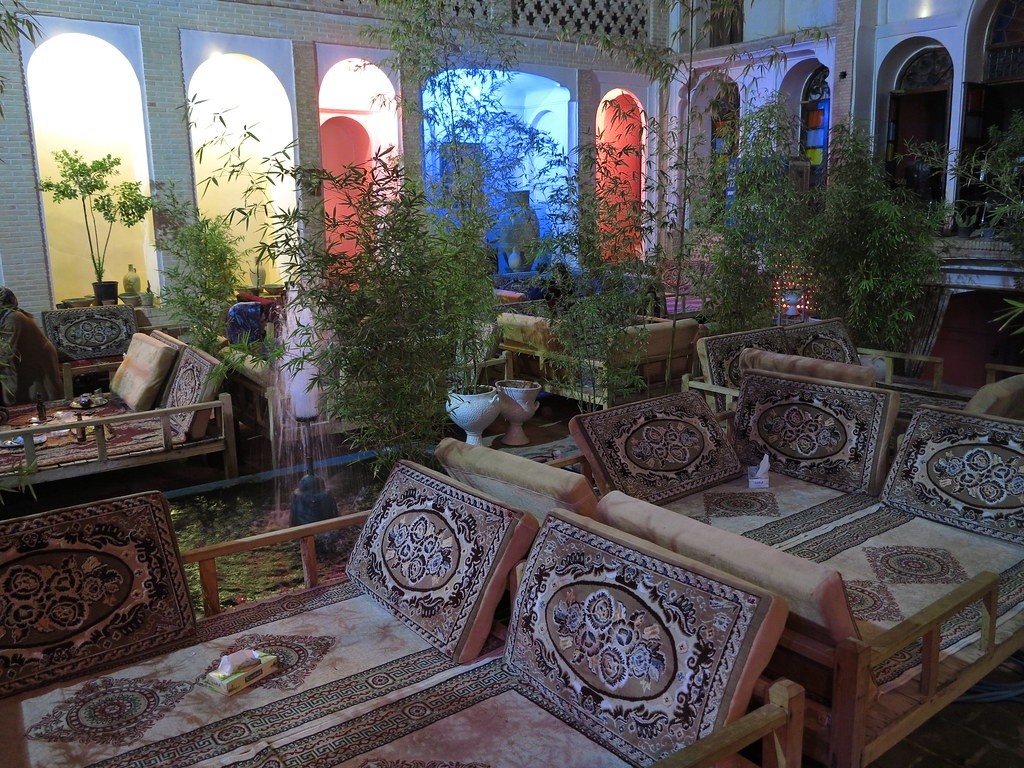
355, 0, 528, 447
36, 149, 157, 305
139, 291, 154, 306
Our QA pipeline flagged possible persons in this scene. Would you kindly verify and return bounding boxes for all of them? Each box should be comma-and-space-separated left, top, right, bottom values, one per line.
0, 285, 62, 407
226, 301, 274, 360
525, 263, 575, 301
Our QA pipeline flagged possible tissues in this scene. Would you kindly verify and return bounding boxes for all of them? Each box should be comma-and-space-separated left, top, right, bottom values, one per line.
747, 452, 771, 489
205, 647, 279, 697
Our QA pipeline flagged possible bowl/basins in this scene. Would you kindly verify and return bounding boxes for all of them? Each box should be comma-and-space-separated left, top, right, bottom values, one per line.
61, 297, 95, 308
84, 294, 96, 306
118, 293, 141, 307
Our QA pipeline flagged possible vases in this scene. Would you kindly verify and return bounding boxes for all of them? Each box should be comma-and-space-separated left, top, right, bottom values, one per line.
498, 190, 540, 271
122, 263, 141, 291
118, 295, 141, 306
495, 379, 542, 446
62, 298, 95, 307
102, 300, 115, 305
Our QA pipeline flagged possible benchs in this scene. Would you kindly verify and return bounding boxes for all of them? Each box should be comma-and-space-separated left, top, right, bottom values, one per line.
0, 271, 1024, 768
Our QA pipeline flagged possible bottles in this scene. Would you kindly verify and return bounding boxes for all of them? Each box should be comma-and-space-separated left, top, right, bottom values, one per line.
76, 412, 85, 441
123, 264, 141, 291
36, 391, 47, 421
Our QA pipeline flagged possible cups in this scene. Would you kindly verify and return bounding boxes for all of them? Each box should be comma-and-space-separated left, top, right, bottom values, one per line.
0, 425, 11, 447
141, 294, 154, 305
102, 300, 115, 306
56, 304, 68, 309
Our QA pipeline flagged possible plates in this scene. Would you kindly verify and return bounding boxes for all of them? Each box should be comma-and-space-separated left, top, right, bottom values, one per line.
51, 410, 77, 417
71, 425, 96, 435
70, 397, 108, 409
0, 434, 47, 448
28, 416, 55, 423
